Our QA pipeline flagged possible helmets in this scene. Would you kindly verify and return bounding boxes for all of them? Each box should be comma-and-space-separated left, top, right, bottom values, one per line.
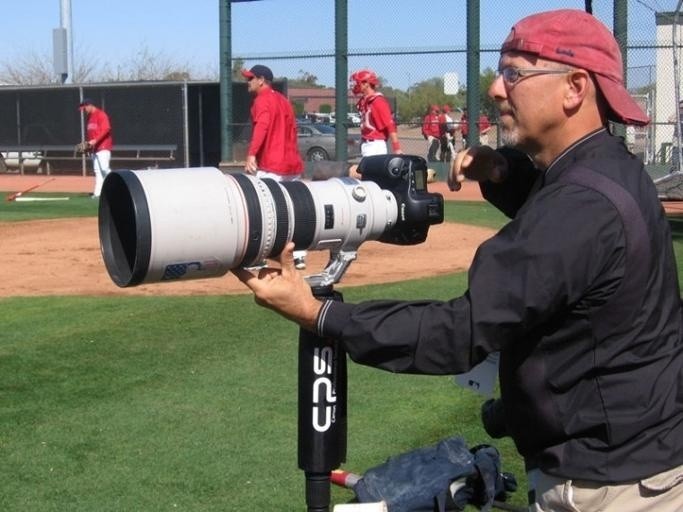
349, 71, 379, 94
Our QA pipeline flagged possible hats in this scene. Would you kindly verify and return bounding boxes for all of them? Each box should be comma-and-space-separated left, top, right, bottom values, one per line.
240, 65, 273, 81
500, 9, 651, 126
431, 104, 451, 113
77, 98, 93, 110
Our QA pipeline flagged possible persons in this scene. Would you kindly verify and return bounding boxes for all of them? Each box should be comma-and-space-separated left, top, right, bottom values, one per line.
78, 98, 113, 200
239, 65, 308, 269
349, 69, 402, 157
424, 103, 490, 163
229, 8, 683, 512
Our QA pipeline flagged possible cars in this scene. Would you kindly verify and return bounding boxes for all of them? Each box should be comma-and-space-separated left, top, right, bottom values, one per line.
0, 152, 43, 173
295, 112, 362, 163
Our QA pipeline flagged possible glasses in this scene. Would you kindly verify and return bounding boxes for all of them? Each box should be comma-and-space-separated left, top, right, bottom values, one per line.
494, 67, 572, 83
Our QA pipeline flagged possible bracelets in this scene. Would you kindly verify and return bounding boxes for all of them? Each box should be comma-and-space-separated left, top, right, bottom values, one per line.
391, 141, 401, 153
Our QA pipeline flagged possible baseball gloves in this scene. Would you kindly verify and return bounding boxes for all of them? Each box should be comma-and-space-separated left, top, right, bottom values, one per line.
76, 141, 92, 154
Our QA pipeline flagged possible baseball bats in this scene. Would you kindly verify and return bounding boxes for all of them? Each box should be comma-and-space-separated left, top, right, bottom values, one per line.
7, 178, 54, 201
